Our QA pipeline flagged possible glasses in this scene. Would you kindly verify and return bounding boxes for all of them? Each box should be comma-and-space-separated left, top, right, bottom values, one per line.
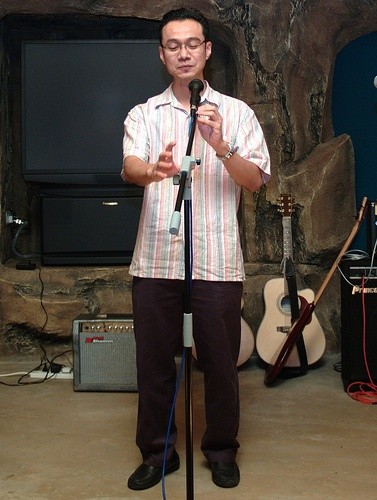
160, 40, 206, 53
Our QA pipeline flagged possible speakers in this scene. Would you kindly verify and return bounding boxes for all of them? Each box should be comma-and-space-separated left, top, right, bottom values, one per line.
340, 257, 377, 393
71, 313, 139, 392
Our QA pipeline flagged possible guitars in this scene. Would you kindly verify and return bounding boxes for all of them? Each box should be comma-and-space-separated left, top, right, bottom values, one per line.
192, 297, 255, 369
256, 193, 325, 370
263, 196, 368, 387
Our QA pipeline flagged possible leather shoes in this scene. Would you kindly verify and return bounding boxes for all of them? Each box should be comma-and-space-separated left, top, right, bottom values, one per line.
208, 461, 240, 488
128, 462, 180, 490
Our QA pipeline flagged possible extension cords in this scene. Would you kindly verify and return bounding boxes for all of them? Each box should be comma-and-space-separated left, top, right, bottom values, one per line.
29, 370, 74, 379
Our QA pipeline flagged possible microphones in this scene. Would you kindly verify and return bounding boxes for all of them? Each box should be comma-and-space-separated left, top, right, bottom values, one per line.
187, 79, 204, 113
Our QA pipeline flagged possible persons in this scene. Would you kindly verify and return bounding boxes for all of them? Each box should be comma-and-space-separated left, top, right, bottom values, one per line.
122, 9, 271, 490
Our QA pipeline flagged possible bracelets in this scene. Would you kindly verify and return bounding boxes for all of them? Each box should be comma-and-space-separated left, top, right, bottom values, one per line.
216, 142, 233, 160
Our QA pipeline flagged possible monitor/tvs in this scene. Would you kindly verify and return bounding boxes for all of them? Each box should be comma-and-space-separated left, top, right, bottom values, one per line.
19, 39, 172, 186
